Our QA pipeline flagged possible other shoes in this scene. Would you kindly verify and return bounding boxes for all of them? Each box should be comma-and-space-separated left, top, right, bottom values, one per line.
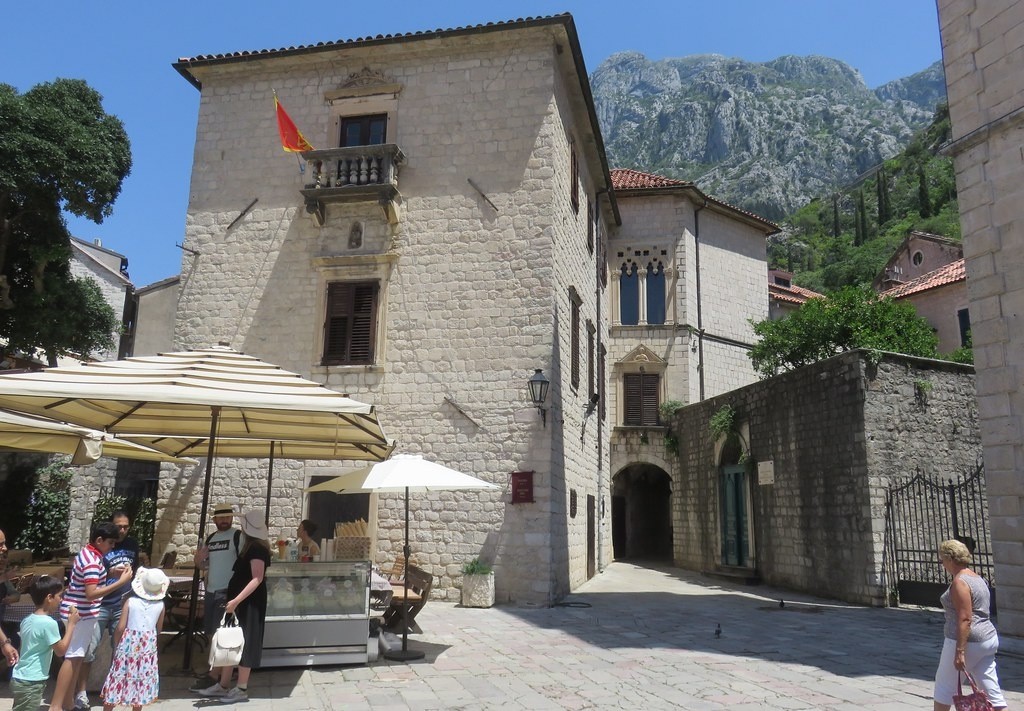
75, 693, 90, 711
189, 675, 218, 690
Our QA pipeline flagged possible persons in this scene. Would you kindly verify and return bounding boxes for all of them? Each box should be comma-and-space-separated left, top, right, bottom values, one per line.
934, 540, 1009, 711
292, 520, 320, 594
0, 529, 19, 667
49, 508, 141, 711
10, 576, 80, 711
188, 503, 271, 702
99, 566, 169, 710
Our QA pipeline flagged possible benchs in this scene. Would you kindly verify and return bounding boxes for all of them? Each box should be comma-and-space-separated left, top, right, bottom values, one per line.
383, 564, 432, 630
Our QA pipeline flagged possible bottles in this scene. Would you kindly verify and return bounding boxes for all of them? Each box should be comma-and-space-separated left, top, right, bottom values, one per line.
278, 540, 298, 563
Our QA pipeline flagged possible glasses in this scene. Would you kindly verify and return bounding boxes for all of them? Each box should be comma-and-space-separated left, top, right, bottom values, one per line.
115, 525, 131, 530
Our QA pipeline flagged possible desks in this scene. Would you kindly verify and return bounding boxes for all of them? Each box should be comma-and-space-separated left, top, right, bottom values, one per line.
161, 566, 206, 579
5, 592, 62, 623
34, 561, 73, 569
14, 564, 64, 582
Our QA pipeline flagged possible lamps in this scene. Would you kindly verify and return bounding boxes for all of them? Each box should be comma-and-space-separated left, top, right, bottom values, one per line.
527, 368, 549, 426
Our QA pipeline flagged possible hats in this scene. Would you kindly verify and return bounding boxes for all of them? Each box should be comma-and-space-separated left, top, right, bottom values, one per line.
131, 566, 170, 600
240, 510, 267, 541
209, 503, 239, 517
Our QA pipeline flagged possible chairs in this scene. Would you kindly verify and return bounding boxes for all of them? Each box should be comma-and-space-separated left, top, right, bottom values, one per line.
374, 555, 410, 607
3, 544, 209, 653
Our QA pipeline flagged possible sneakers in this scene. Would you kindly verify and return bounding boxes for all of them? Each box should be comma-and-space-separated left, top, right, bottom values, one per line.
199, 683, 230, 696
220, 686, 248, 703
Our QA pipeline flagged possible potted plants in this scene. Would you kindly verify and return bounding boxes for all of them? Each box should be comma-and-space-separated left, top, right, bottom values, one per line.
461, 559, 495, 606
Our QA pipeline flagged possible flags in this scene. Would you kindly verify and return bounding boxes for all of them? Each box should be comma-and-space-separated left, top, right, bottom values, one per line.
274, 98, 322, 175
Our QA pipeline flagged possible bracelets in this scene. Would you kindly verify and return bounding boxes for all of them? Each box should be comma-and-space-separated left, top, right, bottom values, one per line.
956, 650, 965, 652
0, 638, 10, 646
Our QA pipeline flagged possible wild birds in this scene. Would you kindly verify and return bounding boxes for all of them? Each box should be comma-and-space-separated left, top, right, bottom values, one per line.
779, 598, 785, 608
715, 623, 722, 639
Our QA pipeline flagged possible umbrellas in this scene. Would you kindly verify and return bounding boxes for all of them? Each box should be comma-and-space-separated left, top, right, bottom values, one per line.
302, 452, 503, 653
0, 339, 397, 669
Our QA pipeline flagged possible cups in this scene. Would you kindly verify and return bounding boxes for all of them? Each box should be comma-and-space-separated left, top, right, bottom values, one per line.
313, 538, 334, 562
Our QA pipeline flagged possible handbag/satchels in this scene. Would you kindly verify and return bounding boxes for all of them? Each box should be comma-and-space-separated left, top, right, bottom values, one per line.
208, 610, 245, 667
953, 667, 994, 711
